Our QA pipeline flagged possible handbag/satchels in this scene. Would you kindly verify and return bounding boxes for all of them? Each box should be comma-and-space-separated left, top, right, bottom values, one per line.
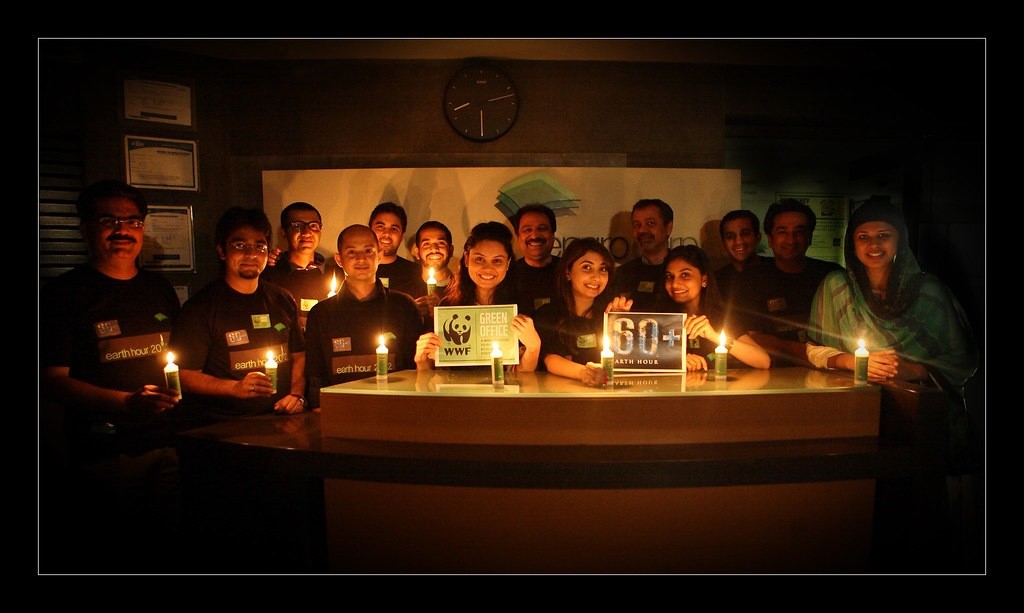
914, 362, 975, 477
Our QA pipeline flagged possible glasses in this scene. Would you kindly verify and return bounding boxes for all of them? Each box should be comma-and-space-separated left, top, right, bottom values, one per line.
226, 241, 268, 253
286, 221, 322, 232
94, 214, 145, 230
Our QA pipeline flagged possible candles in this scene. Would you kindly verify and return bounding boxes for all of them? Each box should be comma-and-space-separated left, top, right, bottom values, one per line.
376, 335, 388, 381
427, 267, 437, 297
327, 270, 337, 298
490, 341, 504, 386
714, 329, 727, 380
854, 340, 869, 385
264, 351, 277, 394
164, 351, 181, 401
600, 335, 615, 386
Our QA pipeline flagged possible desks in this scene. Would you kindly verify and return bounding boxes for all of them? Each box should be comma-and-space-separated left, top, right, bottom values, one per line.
165, 368, 882, 575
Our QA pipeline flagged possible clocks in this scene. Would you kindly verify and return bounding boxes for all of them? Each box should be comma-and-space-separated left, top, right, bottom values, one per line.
444, 63, 519, 144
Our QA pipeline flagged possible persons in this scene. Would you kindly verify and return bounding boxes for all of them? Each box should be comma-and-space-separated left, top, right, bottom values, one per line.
713, 210, 781, 370
731, 197, 847, 366
268, 203, 345, 326
415, 221, 462, 325
265, 202, 427, 300
532, 238, 633, 386
413, 223, 542, 373
613, 199, 680, 312
504, 205, 563, 318
173, 206, 305, 415
603, 245, 771, 370
305, 225, 423, 407
41, 178, 181, 433
806, 202, 981, 412
